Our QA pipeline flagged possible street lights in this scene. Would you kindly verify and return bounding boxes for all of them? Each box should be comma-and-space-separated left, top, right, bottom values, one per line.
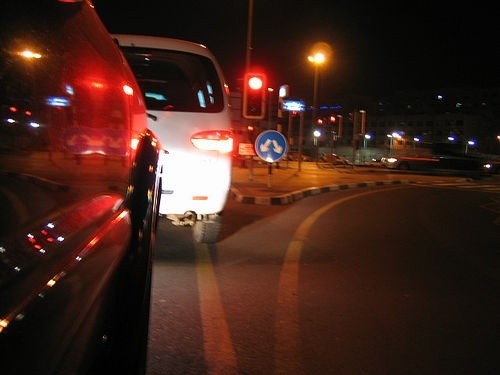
307, 50, 326, 133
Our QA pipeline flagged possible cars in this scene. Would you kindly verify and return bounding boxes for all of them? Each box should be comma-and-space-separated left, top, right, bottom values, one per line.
381, 145, 458, 171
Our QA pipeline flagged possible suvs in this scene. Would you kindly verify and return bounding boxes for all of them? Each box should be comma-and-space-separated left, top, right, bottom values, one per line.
0, 0, 161, 375
109, 32, 236, 245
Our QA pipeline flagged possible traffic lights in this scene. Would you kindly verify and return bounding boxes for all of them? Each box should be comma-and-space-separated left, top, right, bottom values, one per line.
243, 73, 267, 120
